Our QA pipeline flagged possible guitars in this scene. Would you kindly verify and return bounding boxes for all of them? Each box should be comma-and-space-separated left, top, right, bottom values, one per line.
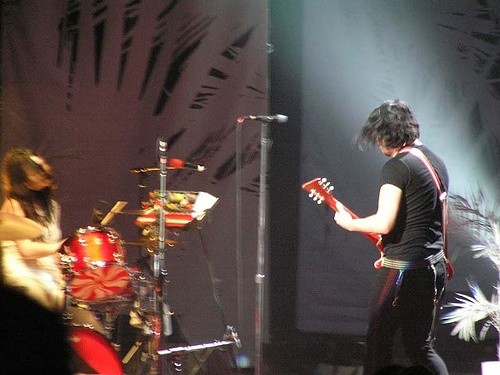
303, 179, 454, 281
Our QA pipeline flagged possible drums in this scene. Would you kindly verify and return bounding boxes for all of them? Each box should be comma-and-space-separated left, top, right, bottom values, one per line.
65, 306, 125, 375
58, 227, 134, 304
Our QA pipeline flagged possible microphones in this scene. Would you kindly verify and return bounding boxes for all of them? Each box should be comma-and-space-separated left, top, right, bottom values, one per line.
243, 114, 290, 123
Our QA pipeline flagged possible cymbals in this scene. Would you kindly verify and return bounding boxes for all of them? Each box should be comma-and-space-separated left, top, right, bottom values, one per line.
134, 165, 206, 174
127, 238, 175, 246
115, 208, 194, 215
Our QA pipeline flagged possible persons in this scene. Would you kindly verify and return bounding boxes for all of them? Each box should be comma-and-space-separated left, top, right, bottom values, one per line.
0, 149, 68, 278
334, 100, 449, 375
